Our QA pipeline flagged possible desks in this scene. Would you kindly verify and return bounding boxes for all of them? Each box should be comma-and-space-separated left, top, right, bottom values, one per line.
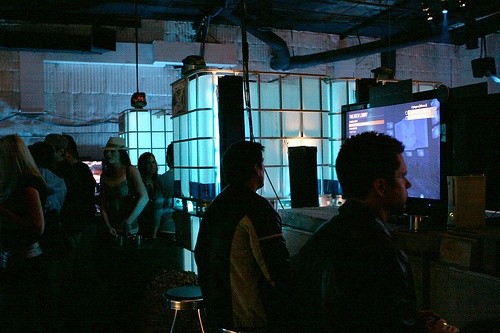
277, 204, 500, 333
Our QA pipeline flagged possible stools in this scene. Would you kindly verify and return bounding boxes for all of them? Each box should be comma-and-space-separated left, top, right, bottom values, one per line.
164, 285, 205, 333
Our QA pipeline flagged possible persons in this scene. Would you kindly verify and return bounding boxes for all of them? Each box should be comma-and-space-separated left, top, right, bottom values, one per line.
289, 131, 456, 333
159, 143, 176, 245
129, 152, 165, 244
100, 136, 150, 246
193, 140, 292, 333
0, 132, 98, 273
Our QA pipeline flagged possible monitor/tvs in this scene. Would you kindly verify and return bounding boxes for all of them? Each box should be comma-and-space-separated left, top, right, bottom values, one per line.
341, 88, 448, 235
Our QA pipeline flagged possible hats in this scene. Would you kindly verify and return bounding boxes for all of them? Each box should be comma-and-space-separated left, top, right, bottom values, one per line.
102, 137, 128, 150
44, 134, 68, 148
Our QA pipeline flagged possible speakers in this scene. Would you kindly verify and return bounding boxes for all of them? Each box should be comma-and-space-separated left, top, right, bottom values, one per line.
288, 146, 319, 208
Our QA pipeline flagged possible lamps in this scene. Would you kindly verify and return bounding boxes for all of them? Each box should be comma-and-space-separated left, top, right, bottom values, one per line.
442, 9, 449, 15
422, 7, 434, 22
